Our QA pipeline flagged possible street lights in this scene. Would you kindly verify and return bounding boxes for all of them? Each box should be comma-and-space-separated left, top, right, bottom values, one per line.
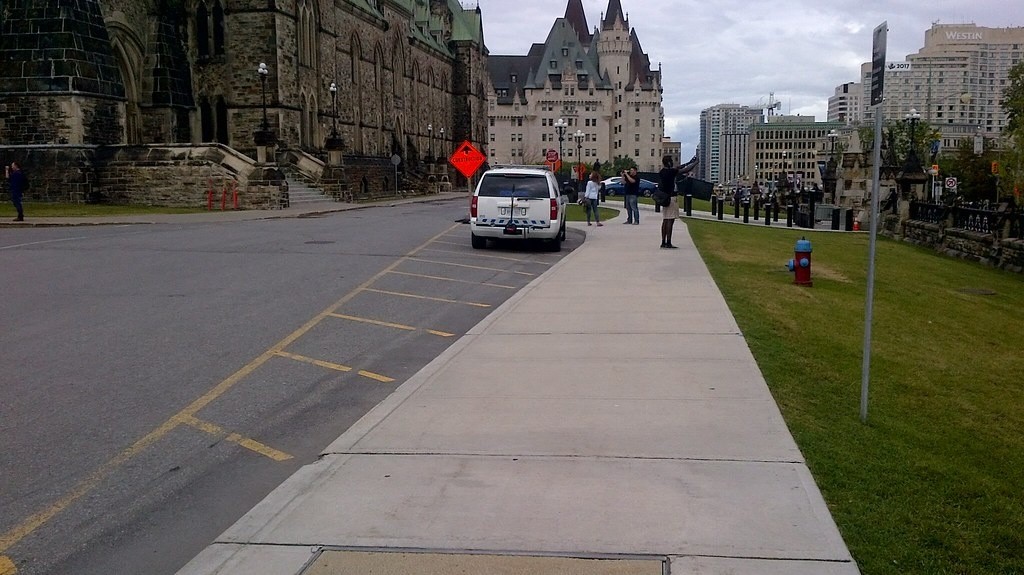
905, 108, 922, 149
573, 129, 585, 182
553, 116, 568, 200
718, 149, 788, 198
255, 63, 275, 131
426, 123, 445, 157
329, 82, 337, 138
827, 129, 839, 159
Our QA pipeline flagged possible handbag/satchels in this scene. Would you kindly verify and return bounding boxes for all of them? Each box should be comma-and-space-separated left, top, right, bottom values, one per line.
652, 188, 671, 207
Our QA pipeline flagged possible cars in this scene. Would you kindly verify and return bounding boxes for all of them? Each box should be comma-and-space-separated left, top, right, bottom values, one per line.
603, 179, 659, 199
599, 176, 624, 187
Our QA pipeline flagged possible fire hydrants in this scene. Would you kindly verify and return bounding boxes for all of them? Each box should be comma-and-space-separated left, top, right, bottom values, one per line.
785, 234, 813, 287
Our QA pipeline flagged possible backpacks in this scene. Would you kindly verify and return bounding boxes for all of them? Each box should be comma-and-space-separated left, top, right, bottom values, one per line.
20, 176, 29, 191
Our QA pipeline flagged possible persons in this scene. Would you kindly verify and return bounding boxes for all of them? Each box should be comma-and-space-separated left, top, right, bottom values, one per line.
657, 153, 699, 249
956, 192, 965, 207
620, 166, 641, 226
593, 158, 601, 173
583, 171, 604, 227
4, 161, 26, 222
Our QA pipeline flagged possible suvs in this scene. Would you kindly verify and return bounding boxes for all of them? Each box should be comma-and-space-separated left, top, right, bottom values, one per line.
470, 164, 568, 253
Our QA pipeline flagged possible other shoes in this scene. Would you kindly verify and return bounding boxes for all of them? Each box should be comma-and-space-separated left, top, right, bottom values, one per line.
13, 218, 24, 221
623, 220, 639, 225
660, 244, 678, 249
587, 223, 603, 226
